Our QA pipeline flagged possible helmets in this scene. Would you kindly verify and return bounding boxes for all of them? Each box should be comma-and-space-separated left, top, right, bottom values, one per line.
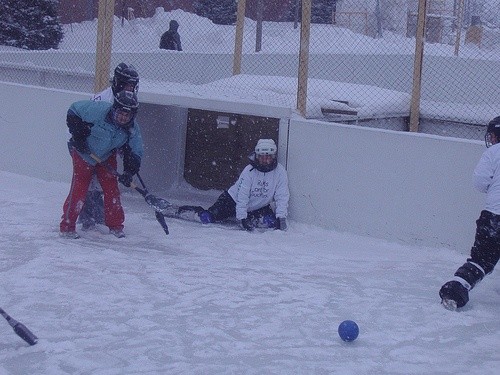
112, 90, 138, 127
485, 116, 500, 148
112, 63, 139, 94
255, 139, 277, 167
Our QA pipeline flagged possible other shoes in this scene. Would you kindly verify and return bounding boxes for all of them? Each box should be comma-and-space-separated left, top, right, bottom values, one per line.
441, 297, 457, 311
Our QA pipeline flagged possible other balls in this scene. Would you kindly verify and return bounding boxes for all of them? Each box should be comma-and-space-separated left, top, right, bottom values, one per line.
338, 320, 359, 341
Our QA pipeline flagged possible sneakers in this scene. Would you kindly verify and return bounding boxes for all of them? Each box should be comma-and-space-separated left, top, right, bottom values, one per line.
108, 228, 125, 239
78, 216, 94, 233
61, 230, 79, 238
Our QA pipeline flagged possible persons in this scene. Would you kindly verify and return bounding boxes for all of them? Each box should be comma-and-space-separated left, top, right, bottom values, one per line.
152, 138, 289, 233
77, 63, 139, 232
58, 90, 142, 240
160, 20, 182, 51
439, 115, 500, 308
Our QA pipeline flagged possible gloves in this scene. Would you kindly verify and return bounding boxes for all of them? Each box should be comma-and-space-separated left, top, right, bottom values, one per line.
124, 152, 141, 175
239, 219, 253, 232
275, 216, 286, 231
71, 132, 91, 155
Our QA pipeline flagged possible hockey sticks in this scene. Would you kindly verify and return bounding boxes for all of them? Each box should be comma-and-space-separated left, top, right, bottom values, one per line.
134, 167, 169, 235
0, 307, 39, 345
67, 140, 146, 197
